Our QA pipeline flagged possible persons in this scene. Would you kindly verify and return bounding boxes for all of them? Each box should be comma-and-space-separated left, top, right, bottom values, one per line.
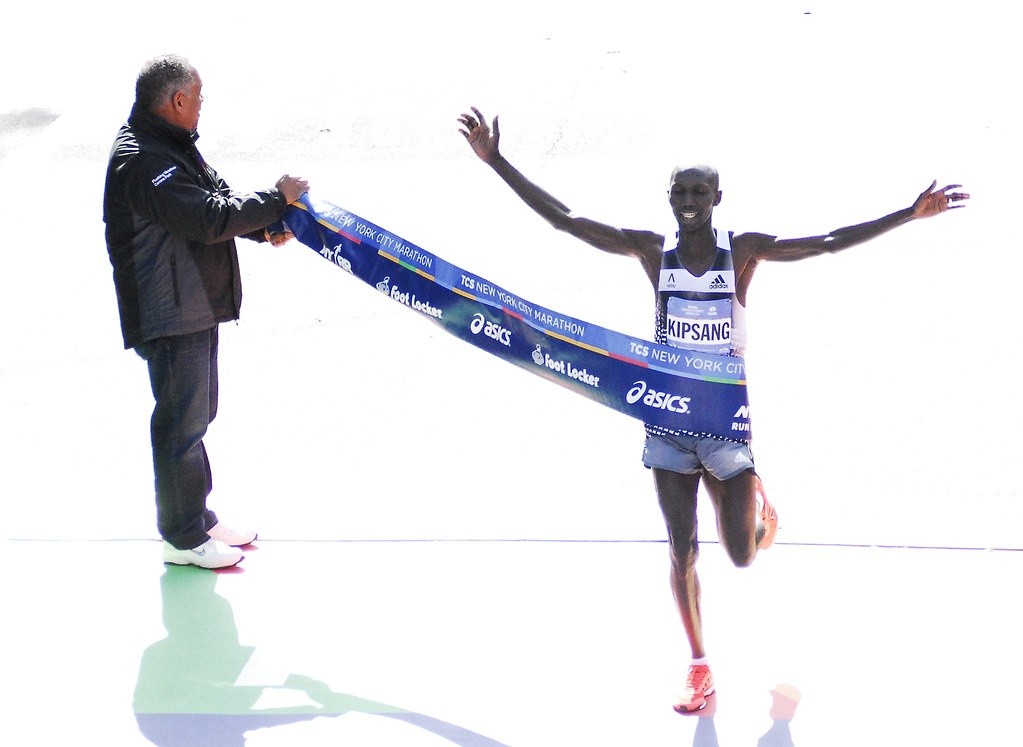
101, 55, 310, 571
457, 108, 970, 714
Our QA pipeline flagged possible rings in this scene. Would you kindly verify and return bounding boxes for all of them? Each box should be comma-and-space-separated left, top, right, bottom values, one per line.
948, 199, 952, 203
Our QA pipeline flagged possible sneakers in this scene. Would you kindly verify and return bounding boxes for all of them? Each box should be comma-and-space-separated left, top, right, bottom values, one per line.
672, 665, 715, 712
207, 521, 258, 546
163, 538, 244, 569
755, 473, 778, 551
